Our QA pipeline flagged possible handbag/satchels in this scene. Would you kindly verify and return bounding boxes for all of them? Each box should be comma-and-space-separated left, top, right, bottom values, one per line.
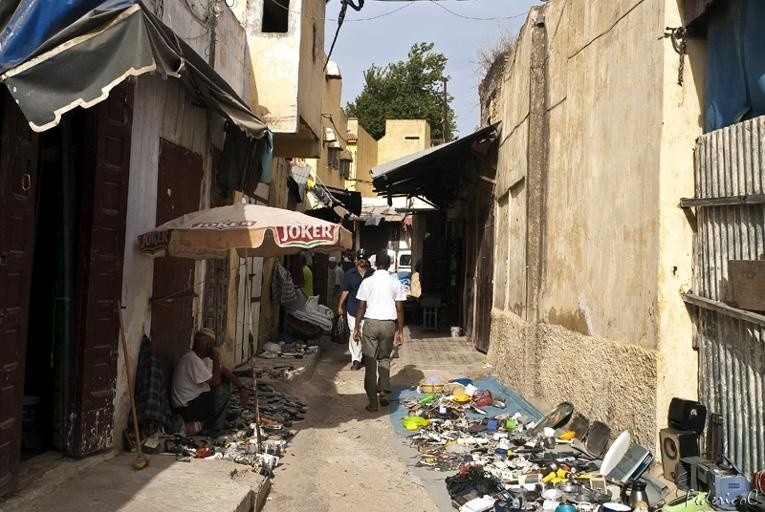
330, 314, 349, 344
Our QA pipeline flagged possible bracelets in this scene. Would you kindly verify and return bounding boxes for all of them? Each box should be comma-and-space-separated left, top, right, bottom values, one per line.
239, 386, 245, 390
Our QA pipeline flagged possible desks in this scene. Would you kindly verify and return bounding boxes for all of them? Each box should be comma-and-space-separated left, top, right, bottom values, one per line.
420, 292, 442, 331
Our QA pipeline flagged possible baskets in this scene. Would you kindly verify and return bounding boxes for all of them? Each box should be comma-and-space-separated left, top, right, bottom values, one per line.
419, 378, 448, 393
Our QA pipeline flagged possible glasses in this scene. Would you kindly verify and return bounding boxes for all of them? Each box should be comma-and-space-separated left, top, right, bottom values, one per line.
358, 257, 365, 260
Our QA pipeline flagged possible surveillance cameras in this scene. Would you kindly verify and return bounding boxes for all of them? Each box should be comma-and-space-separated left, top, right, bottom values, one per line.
536, 17, 546, 28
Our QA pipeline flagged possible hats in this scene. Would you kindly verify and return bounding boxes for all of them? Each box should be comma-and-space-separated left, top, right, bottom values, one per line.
199, 328, 214, 342
356, 249, 368, 258
329, 257, 337, 262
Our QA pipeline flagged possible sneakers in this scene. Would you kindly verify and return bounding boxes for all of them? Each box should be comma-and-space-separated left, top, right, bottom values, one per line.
366, 404, 378, 412
263, 353, 277, 358
380, 392, 390, 406
351, 361, 360, 371
257, 351, 271, 357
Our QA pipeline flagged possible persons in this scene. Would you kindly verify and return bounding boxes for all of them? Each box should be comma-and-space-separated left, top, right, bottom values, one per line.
289, 249, 423, 411
170, 328, 249, 437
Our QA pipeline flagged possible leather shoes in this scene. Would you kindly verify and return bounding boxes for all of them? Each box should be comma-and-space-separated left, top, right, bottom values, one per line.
164, 439, 189, 457
173, 435, 198, 448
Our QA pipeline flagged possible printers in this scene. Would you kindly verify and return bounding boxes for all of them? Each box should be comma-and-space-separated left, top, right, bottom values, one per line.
699, 463, 748, 511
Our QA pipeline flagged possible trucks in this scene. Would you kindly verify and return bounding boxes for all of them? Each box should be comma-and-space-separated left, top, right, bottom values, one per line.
366, 248, 411, 273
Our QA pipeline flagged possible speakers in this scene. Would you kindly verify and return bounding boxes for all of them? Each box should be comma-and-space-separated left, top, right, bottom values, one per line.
659, 430, 696, 482
667, 398, 706, 434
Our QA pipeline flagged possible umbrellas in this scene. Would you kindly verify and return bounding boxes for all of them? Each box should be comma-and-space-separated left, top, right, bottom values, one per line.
138, 196, 353, 422
